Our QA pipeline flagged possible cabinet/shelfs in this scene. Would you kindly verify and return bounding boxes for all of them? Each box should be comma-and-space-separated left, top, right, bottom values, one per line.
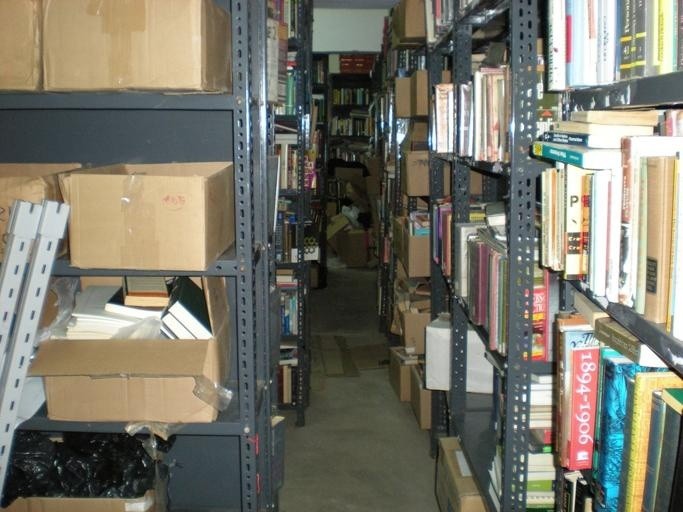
1, 1, 313, 511
426, 1, 683, 508
313, 49, 386, 333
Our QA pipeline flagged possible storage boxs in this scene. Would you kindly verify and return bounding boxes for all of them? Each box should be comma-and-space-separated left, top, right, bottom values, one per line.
2, 1, 41, 95
58, 161, 234, 271
27, 273, 234, 423
386, 0, 434, 430
1, 162, 81, 263
41, 0, 233, 96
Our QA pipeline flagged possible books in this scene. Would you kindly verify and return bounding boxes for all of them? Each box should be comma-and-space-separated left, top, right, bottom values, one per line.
68, 275, 211, 341
431, 0, 682, 510
378, 48, 429, 365
316, 53, 375, 199
268, 1, 320, 402
